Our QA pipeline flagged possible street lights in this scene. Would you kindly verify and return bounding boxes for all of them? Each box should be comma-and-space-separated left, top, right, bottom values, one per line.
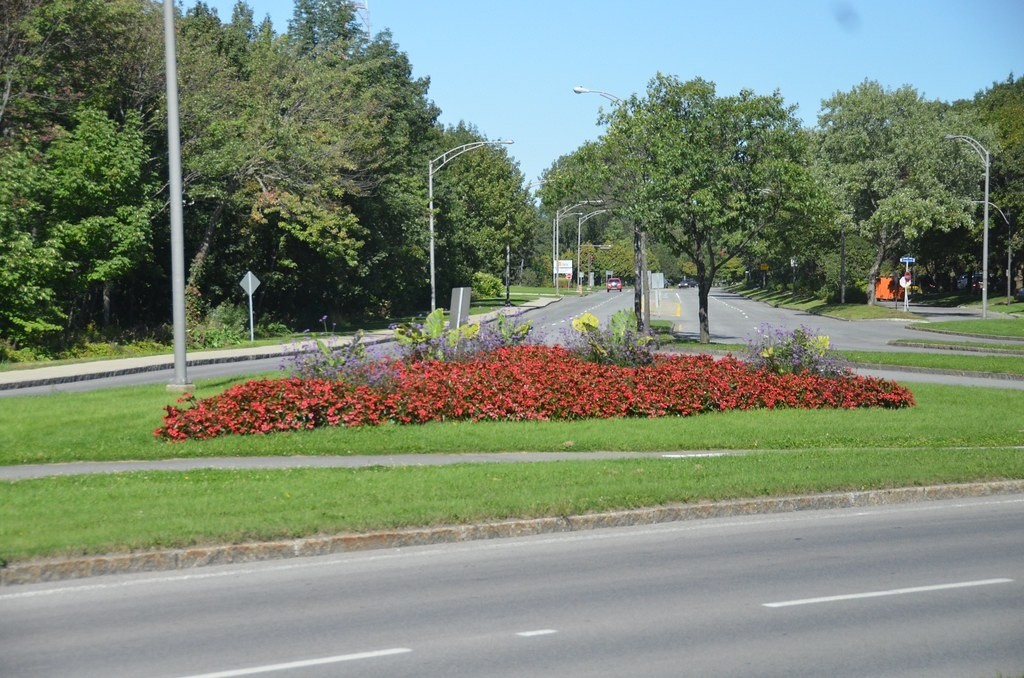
944, 132, 989, 319
574, 207, 620, 290
429, 138, 516, 312
555, 198, 607, 296
574, 84, 652, 333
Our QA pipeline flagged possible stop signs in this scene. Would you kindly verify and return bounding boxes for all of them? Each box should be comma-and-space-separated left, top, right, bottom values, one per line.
905, 271, 912, 282
